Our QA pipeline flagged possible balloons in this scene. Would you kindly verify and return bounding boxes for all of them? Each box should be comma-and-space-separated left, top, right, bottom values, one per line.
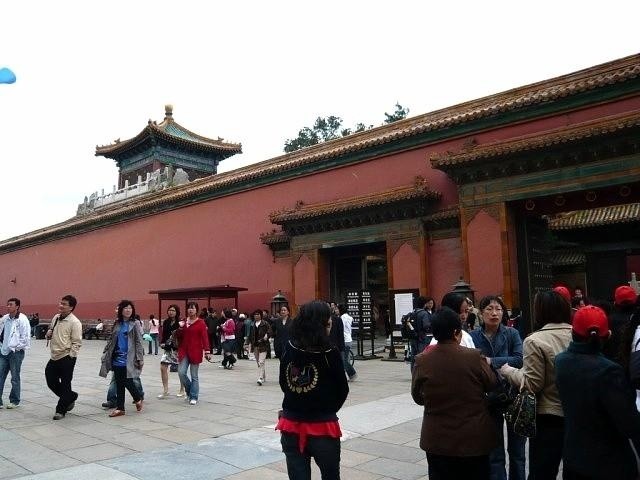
0, 65, 17, 87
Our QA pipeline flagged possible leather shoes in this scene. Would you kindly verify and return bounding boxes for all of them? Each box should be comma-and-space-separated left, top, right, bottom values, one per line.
53, 393, 78, 419
102, 401, 142, 417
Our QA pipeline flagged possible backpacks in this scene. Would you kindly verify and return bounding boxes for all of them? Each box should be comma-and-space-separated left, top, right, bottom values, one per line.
401, 309, 423, 350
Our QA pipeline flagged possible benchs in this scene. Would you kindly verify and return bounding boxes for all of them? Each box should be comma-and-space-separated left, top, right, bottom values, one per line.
82, 323, 116, 340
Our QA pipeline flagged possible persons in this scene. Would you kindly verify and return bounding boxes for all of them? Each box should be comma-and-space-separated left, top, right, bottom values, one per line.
44, 293, 84, 420
277, 300, 351, 479
27, 309, 40, 339
0, 296, 31, 411
89, 301, 357, 416
406, 285, 640, 479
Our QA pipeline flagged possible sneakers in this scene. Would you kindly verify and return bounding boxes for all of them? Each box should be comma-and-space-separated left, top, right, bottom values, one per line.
7, 403, 19, 408
177, 393, 196, 404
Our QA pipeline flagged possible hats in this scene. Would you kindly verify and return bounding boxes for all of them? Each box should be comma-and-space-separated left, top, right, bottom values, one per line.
573, 306, 608, 337
554, 287, 570, 304
615, 286, 637, 304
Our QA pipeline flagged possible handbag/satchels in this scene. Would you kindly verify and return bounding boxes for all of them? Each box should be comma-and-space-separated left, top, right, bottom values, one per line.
506, 376, 536, 437
